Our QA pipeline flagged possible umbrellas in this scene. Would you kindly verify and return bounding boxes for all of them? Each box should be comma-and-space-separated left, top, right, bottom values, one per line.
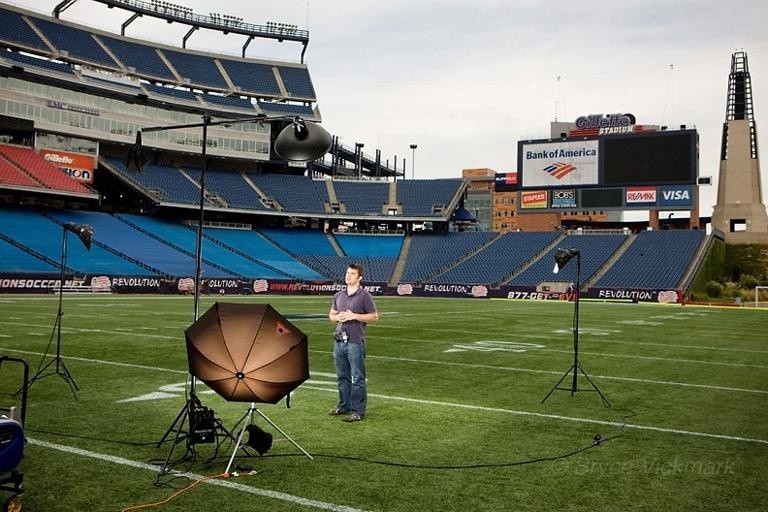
182, 301, 310, 407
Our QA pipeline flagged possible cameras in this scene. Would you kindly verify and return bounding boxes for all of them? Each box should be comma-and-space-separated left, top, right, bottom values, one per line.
335, 331, 350, 343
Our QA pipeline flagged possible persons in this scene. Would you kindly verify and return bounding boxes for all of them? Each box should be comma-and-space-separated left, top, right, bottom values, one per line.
327, 264, 378, 423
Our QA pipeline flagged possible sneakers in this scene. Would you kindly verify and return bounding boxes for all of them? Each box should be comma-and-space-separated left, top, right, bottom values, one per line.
328, 408, 345, 416
343, 412, 363, 422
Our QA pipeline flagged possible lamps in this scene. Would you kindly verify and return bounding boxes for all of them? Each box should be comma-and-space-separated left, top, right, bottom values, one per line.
270, 112, 336, 165
14, 218, 98, 402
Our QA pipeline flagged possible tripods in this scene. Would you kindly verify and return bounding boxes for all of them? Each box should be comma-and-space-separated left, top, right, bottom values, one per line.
156, 376, 251, 473
214, 403, 313, 474
11, 233, 79, 400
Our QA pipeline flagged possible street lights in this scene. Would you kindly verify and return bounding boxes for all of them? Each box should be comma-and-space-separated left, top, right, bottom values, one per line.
410, 144, 418, 180
354, 142, 364, 179
